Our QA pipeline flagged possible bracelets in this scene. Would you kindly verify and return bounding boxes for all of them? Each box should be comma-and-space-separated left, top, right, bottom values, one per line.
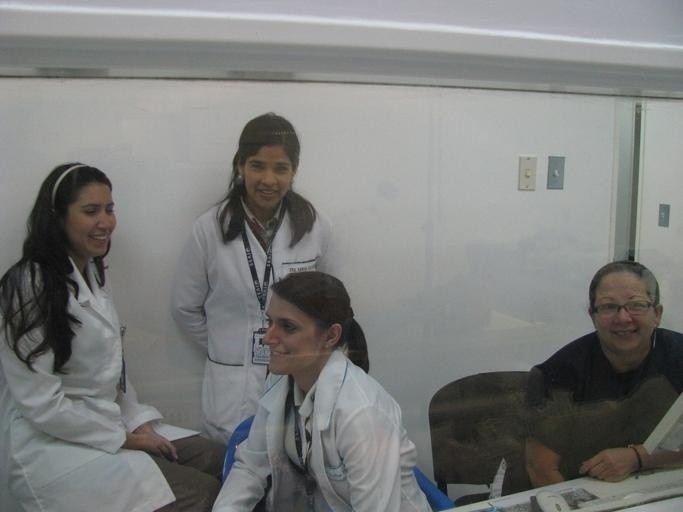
625, 444, 642, 471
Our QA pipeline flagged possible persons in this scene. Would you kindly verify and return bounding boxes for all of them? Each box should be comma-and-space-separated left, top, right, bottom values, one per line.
168, 113, 329, 446
209, 270, 433, 512
521, 261, 682, 489
0, 162, 230, 512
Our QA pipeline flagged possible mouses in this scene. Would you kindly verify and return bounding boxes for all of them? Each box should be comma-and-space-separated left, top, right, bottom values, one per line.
536, 490, 570, 512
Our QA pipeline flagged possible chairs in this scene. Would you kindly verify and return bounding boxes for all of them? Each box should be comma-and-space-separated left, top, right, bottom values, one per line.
428, 370, 546, 506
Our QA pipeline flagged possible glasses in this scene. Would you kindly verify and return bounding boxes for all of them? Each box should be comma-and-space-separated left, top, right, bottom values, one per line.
591, 300, 658, 316
